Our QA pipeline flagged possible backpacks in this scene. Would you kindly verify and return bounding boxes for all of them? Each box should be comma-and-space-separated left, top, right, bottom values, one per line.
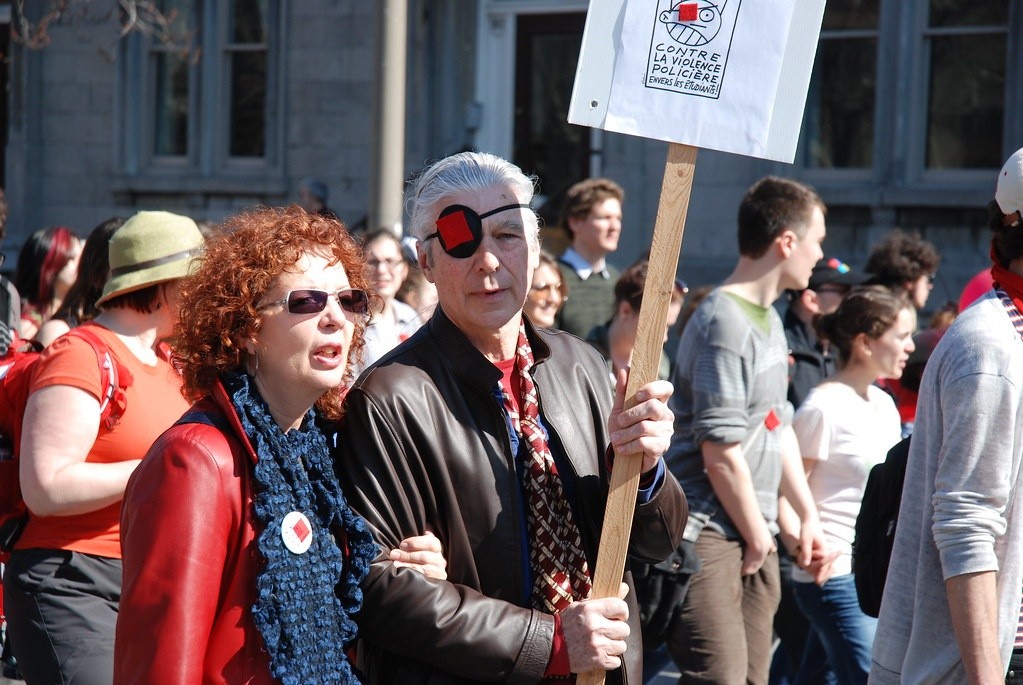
0, 326, 132, 551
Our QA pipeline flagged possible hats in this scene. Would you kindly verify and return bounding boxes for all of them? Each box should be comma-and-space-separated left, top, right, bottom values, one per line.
994, 147, 1023, 227
785, 258, 877, 302
94, 211, 206, 309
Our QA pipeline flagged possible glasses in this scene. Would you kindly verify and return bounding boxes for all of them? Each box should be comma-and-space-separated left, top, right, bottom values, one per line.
924, 272, 937, 282
255, 289, 369, 314
815, 284, 852, 296
368, 258, 406, 267
530, 282, 570, 305
631, 276, 689, 300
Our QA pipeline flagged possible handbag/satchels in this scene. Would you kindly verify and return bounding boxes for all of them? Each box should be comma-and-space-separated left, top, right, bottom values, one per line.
851, 433, 913, 620
631, 529, 700, 636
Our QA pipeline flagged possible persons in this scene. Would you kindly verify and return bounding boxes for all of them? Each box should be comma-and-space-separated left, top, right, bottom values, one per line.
547, 176, 630, 367
330, 151, 691, 685
770, 237, 962, 685
521, 247, 564, 330
112, 204, 448, 685
588, 257, 684, 384
0, 185, 213, 684
348, 230, 439, 398
662, 174, 831, 685
864, 148, 1023, 685
